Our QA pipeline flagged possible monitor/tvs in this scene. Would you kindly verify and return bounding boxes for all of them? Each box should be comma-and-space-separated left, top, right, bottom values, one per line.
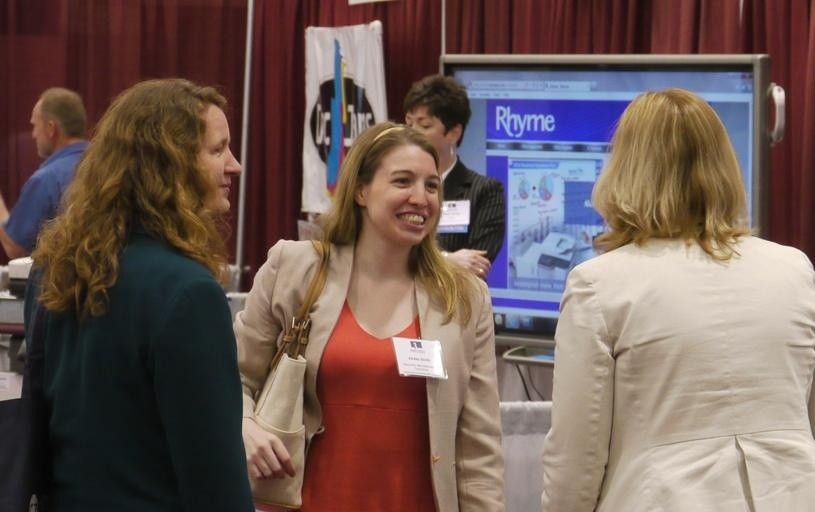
440, 52, 767, 351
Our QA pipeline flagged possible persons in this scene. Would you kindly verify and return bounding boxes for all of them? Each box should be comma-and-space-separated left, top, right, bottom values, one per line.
536, 88, 815, 511
0, 86, 95, 259
231, 121, 510, 512
20, 78, 257, 512
400, 75, 507, 283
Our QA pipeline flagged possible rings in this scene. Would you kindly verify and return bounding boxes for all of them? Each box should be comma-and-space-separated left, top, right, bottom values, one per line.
478, 268, 484, 274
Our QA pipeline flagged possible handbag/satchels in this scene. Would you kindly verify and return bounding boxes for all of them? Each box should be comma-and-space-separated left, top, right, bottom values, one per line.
245, 352, 308, 510
